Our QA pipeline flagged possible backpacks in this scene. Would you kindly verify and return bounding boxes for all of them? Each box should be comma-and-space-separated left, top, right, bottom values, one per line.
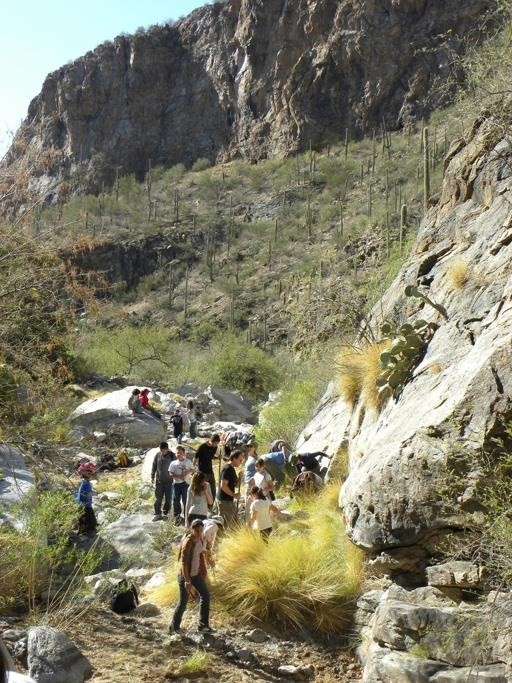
95, 447, 131, 471
108, 578, 138, 613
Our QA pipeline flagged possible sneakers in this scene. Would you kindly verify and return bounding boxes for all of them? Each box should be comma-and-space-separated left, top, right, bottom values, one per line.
196, 627, 217, 634
152, 515, 182, 526
169, 628, 187, 635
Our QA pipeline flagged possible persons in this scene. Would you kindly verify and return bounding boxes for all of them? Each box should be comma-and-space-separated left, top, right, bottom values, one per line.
140, 388, 163, 417
169, 408, 183, 437
151, 432, 334, 634
185, 400, 197, 438
127, 388, 145, 415
76, 461, 99, 535
